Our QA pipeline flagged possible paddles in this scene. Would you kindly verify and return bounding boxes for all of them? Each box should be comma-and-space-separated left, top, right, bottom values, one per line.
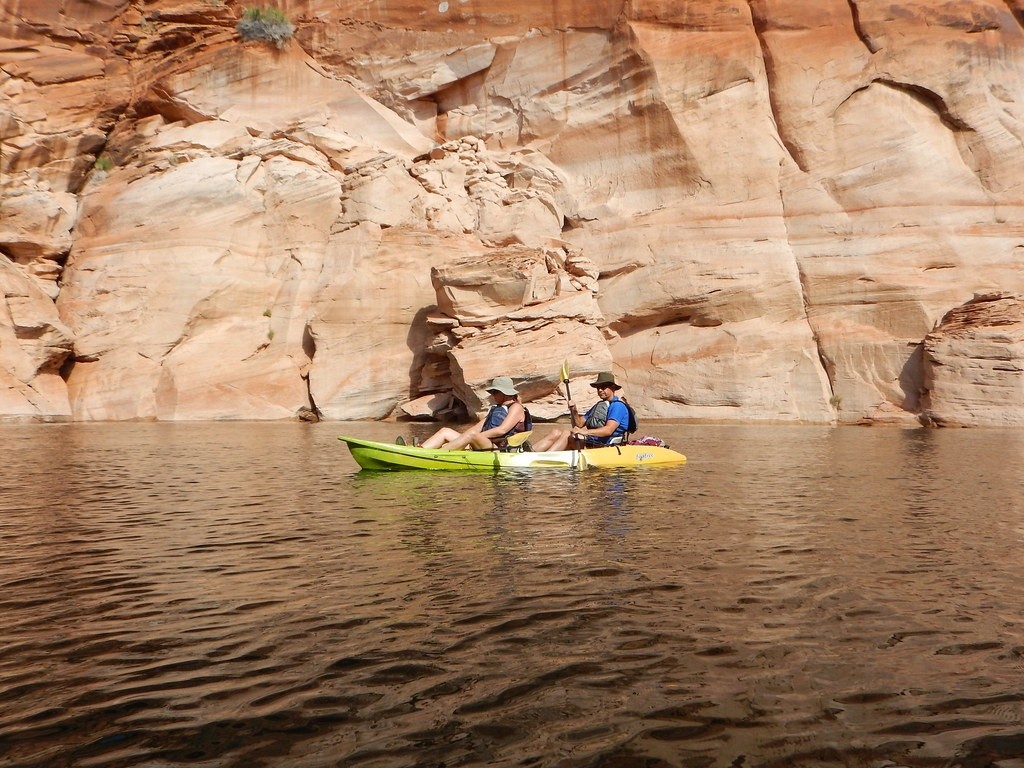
490, 430, 534, 447
559, 359, 589, 472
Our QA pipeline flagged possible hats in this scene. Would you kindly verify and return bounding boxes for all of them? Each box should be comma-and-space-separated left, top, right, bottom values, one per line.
590, 372, 621, 391
485, 377, 519, 396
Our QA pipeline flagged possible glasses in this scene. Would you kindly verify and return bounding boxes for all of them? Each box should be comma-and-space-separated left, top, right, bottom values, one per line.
490, 390, 499, 395
597, 384, 612, 390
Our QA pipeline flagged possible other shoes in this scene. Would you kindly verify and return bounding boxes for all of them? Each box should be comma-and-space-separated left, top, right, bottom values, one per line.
522, 441, 535, 452
516, 447, 520, 453
396, 436, 407, 446
413, 436, 423, 448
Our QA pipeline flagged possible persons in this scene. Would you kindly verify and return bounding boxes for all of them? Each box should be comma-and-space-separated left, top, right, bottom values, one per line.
396, 377, 525, 453
522, 372, 629, 452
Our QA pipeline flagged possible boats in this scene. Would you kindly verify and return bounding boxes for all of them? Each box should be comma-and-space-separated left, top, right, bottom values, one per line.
336, 436, 689, 473
